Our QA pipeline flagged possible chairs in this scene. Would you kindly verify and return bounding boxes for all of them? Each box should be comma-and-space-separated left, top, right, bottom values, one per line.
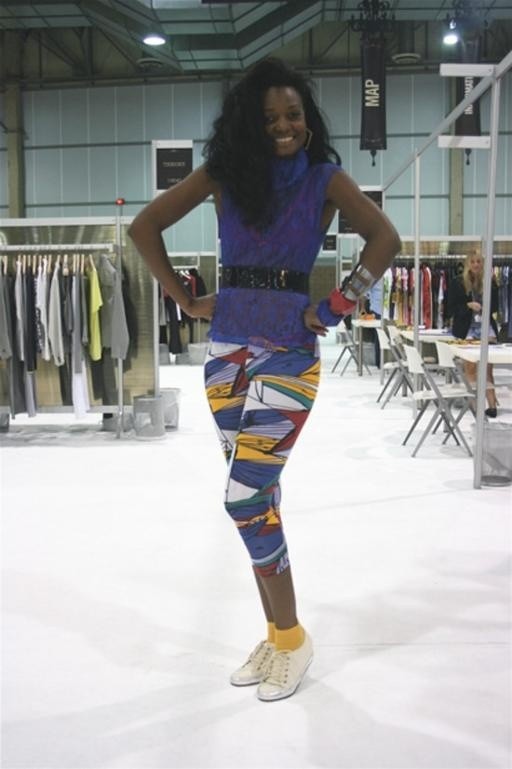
332, 318, 478, 458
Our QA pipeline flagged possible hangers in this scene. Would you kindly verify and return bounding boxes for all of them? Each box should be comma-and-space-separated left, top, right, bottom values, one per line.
174, 265, 198, 280
393, 254, 512, 270
0, 244, 114, 276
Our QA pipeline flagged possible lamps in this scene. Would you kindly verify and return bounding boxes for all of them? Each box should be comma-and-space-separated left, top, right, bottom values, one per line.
143, 32, 167, 46
442, 14, 459, 45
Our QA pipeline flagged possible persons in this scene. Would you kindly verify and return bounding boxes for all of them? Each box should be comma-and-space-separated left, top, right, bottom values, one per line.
444, 248, 500, 419
125, 57, 402, 704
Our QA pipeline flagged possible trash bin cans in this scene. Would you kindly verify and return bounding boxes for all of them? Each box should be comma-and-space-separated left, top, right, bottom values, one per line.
362, 343, 376, 365
133, 388, 181, 440
471, 422, 512, 489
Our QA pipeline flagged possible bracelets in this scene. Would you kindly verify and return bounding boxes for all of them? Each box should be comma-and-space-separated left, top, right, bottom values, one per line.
353, 263, 378, 288
315, 299, 342, 329
346, 273, 368, 297
339, 284, 357, 304
327, 288, 355, 320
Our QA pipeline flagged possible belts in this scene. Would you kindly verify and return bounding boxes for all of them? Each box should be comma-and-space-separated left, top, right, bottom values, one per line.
218, 266, 310, 294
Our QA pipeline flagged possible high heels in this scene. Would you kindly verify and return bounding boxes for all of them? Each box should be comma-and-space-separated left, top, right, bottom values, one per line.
486, 397, 499, 417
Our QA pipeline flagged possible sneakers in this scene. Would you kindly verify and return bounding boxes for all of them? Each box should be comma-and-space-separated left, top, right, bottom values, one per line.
230, 639, 276, 686
256, 630, 314, 701
